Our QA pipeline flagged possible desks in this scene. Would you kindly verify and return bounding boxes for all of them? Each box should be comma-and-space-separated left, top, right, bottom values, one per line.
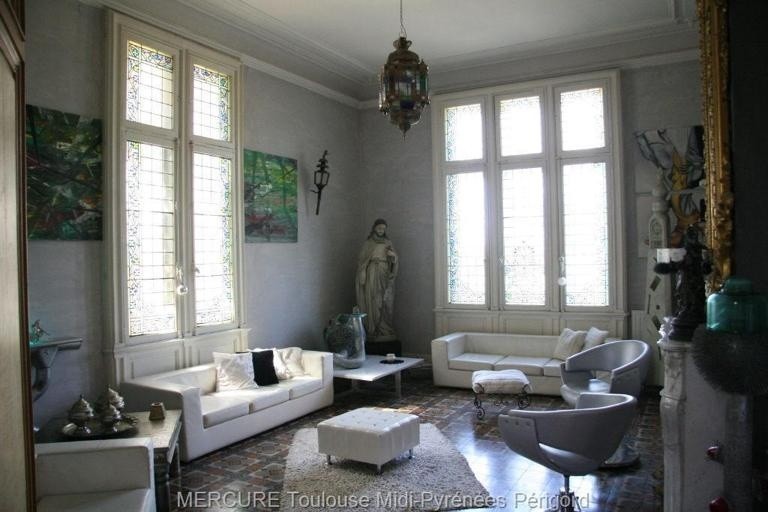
658, 314, 730, 512
55, 409, 183, 512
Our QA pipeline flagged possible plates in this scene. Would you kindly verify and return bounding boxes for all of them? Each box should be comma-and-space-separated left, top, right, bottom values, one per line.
380, 360, 404, 364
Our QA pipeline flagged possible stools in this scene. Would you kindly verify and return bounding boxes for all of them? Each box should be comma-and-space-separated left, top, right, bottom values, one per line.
472, 369, 532, 420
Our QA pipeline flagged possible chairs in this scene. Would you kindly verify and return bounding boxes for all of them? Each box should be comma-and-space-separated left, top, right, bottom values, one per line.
558, 339, 652, 470
34, 438, 157, 512
497, 391, 639, 512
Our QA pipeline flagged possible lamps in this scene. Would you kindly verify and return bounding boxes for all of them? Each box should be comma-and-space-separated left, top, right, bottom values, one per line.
377, 0, 431, 138
309, 149, 331, 215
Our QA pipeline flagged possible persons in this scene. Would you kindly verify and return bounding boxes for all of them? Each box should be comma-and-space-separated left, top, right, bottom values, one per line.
355, 217, 398, 340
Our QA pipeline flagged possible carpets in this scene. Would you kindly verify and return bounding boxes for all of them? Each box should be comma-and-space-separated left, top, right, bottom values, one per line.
280, 423, 495, 512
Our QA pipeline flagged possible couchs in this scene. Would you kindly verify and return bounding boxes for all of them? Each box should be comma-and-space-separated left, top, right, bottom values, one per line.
121, 351, 333, 464
430, 331, 621, 397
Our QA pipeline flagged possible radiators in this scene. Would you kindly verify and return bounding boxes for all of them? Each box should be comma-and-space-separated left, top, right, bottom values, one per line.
631, 310, 668, 387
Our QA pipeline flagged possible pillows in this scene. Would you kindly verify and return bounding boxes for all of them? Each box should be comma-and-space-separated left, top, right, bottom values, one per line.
211, 346, 302, 392
552, 327, 584, 361
580, 326, 607, 352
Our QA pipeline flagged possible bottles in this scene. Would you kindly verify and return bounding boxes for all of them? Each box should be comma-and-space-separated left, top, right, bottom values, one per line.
706, 278, 767, 334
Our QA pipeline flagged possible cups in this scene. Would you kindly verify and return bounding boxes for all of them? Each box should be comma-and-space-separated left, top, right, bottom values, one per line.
386, 354, 395, 362
